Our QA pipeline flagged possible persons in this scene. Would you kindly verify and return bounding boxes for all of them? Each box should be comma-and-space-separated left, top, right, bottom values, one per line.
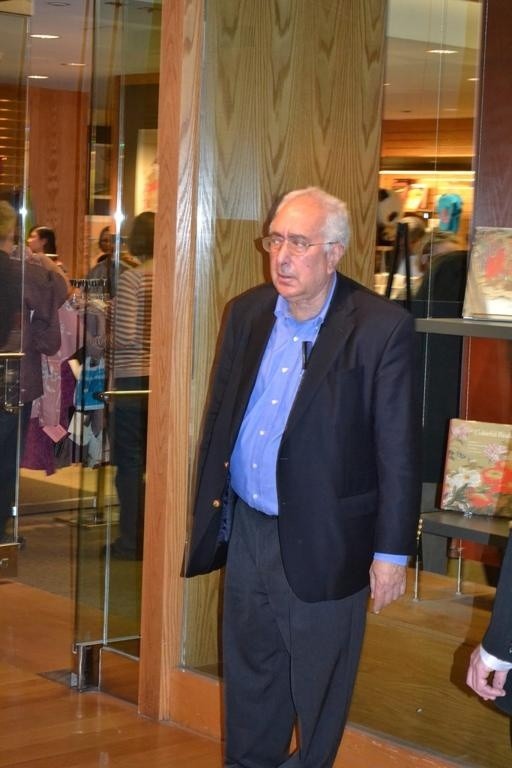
395, 226, 462, 301
27, 222, 59, 260
0, 199, 72, 532
95, 225, 137, 272
183, 182, 429, 767
397, 226, 433, 278
85, 211, 155, 563
464, 523, 510, 723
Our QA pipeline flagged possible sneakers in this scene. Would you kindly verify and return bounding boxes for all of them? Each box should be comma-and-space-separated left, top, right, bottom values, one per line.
101, 541, 143, 561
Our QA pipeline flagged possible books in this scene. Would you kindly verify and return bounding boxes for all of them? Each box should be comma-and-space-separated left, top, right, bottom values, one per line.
458, 226, 512, 324
439, 418, 512, 522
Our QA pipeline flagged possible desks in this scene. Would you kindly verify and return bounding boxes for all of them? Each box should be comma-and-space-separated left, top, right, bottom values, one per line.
413, 510, 512, 601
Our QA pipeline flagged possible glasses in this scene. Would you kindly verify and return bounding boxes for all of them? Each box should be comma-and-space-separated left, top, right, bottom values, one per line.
262, 236, 339, 254
